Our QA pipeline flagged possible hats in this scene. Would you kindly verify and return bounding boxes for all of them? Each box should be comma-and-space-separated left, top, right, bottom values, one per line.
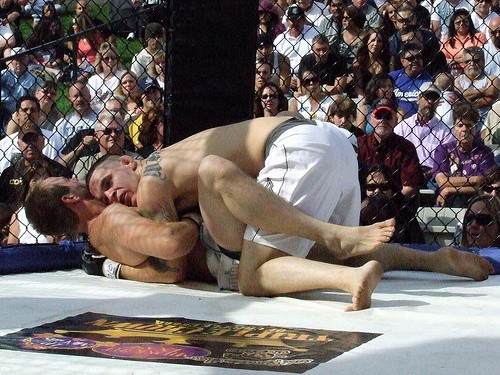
285, 5, 304, 21
418, 82, 441, 99
258, 0, 278, 18
144, 22, 162, 40
137, 76, 160, 95
18, 122, 42, 140
370, 97, 398, 115
10, 47, 29, 57
329, 96, 357, 119
257, 34, 273, 46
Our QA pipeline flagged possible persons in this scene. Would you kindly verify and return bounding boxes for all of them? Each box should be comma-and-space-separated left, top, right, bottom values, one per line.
0, 0, 500, 312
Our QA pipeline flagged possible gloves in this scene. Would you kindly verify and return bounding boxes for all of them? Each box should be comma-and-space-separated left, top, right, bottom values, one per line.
81, 249, 122, 280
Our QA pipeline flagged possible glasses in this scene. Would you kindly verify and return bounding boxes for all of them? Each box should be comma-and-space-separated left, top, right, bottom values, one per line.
330, 109, 354, 117
38, 89, 57, 96
301, 77, 319, 86
396, 18, 410, 23
403, 54, 422, 62
371, 112, 394, 120
464, 213, 497, 226
463, 59, 481, 64
363, 183, 392, 191
101, 56, 118, 61
340, 15, 350, 20
97, 127, 125, 135
260, 94, 279, 99
